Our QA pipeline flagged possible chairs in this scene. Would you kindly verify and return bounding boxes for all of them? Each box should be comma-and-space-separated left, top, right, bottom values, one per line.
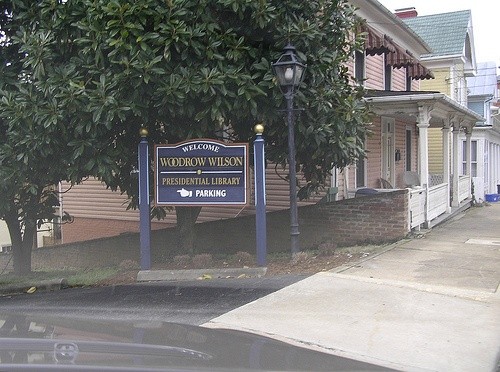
403, 171, 420, 188
380, 177, 395, 189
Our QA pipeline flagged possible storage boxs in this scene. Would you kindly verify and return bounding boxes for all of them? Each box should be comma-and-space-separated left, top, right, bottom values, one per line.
485, 194, 498, 202
496, 194, 500, 201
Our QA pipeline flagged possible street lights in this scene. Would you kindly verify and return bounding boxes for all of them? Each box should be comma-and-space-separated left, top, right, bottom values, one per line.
270, 32, 306, 254
